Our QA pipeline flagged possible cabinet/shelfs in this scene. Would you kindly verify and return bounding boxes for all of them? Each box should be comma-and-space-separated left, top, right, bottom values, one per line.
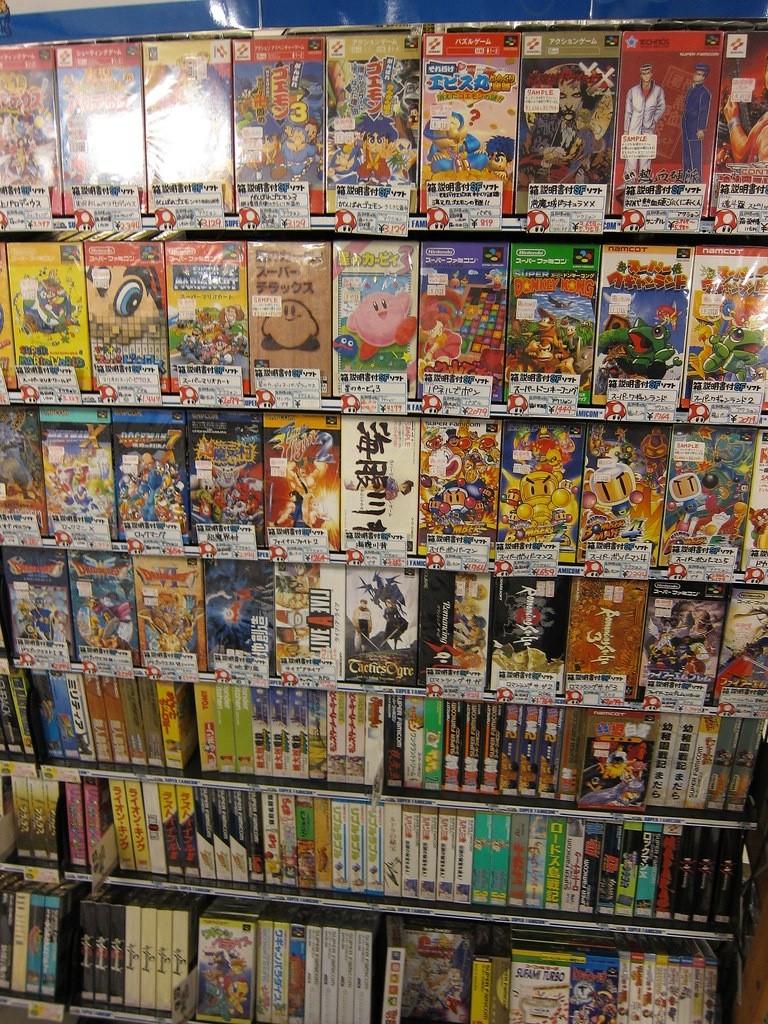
2, 0, 767, 1024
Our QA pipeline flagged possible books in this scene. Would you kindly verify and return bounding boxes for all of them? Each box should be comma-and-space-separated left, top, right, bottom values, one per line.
0, 666, 768, 1024
